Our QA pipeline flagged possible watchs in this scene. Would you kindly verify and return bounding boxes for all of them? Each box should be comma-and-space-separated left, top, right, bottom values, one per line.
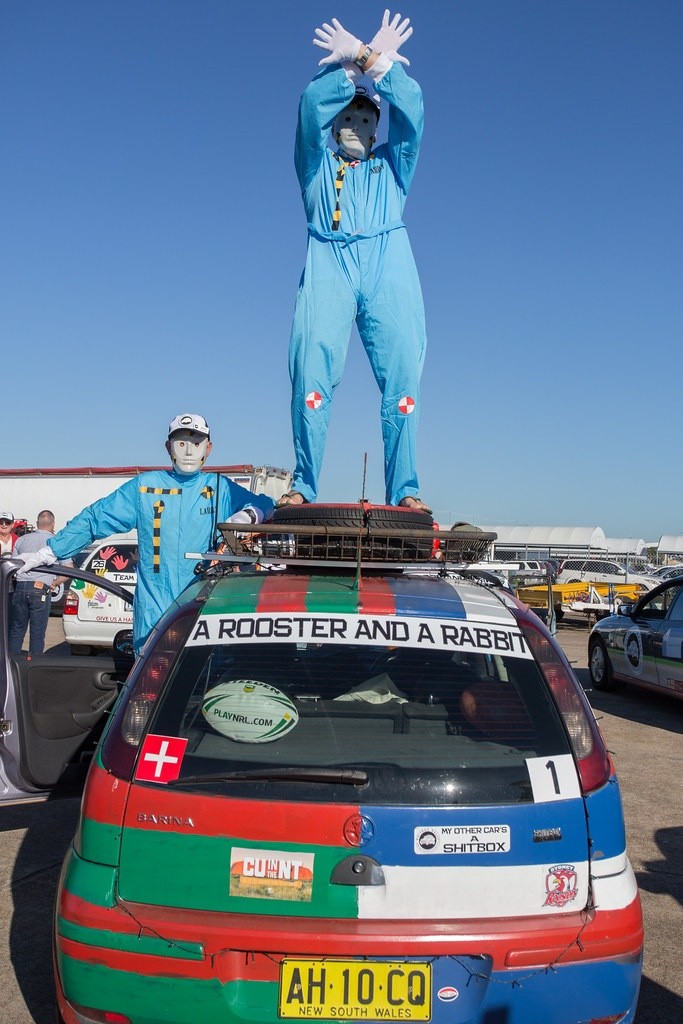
242, 509, 257, 524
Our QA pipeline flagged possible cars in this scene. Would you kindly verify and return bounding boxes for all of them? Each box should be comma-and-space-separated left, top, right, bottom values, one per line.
59, 534, 139, 655
477, 556, 683, 622
584, 575, 683, 700
50, 542, 101, 618
1, 497, 645, 1024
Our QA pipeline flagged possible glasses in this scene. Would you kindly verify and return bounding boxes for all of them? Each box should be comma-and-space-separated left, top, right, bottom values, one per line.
0, 519, 13, 525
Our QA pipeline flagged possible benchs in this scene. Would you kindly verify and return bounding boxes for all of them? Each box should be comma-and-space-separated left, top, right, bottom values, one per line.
188, 702, 490, 737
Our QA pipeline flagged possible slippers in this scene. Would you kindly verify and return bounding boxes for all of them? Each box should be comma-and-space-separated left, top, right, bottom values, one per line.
273, 491, 305, 509
398, 496, 433, 515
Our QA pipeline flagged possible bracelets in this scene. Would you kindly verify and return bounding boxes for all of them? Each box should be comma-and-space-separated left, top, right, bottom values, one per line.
356, 46, 372, 68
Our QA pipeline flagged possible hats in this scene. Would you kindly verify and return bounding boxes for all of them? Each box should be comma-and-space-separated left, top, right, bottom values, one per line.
352, 82, 380, 109
168, 413, 211, 444
0, 511, 13, 520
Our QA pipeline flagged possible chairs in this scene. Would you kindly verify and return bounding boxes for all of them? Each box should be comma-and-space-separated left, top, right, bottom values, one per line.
217, 643, 297, 691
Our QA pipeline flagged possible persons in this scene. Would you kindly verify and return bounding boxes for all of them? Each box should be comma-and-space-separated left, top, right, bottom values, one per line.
0, 510, 75, 657
11, 414, 276, 661
277, 9, 435, 516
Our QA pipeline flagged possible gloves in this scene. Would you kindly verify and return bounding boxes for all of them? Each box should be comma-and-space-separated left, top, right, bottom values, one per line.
367, 9, 414, 67
226, 510, 251, 539
11, 546, 58, 574
312, 18, 362, 66
